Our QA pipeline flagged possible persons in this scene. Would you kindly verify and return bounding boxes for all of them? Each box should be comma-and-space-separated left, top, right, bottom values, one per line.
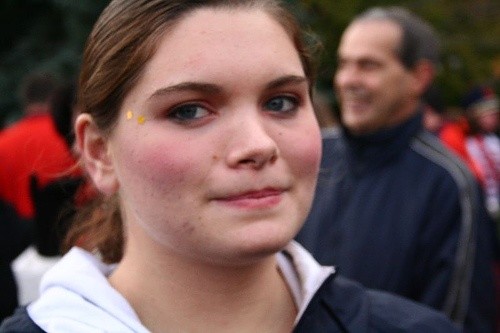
1, 1, 461, 333
293, 8, 492, 333
1, 69, 103, 319
426, 75, 499, 222
